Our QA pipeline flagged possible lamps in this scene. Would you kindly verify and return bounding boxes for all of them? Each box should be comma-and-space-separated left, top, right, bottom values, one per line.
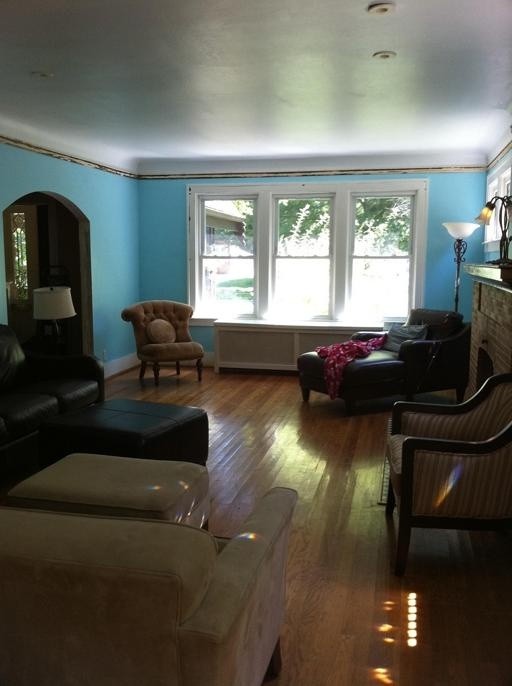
32, 286, 79, 351
440, 222, 481, 312
474, 195, 512, 265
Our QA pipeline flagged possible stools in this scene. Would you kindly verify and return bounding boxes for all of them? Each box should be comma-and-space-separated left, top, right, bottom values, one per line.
5, 397, 212, 535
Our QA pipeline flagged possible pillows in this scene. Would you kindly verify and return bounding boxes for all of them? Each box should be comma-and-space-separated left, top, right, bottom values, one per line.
382, 324, 428, 353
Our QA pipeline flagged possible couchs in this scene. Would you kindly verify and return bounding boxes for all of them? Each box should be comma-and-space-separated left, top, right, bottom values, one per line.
0, 324, 105, 476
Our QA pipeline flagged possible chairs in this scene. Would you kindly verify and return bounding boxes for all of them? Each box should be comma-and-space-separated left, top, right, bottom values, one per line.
295, 320, 471, 418
0, 485, 298, 685
122, 300, 204, 387
385, 372, 512, 578
